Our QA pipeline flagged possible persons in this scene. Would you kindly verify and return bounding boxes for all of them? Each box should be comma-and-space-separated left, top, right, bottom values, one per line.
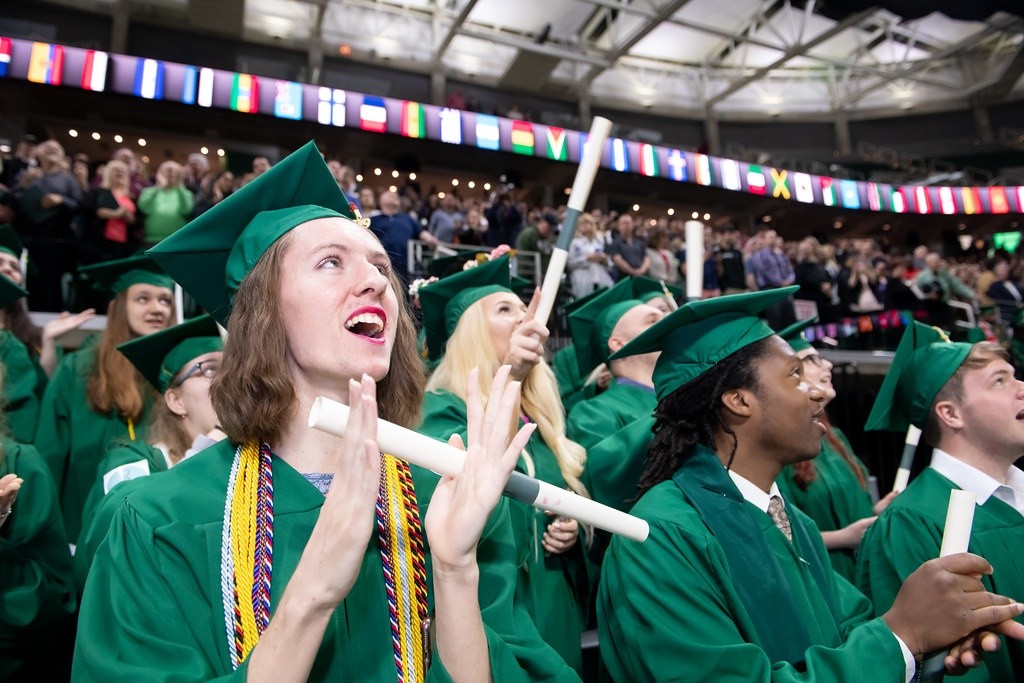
71, 139, 583, 683
412, 243, 593, 678
0, 87, 1024, 580
0, 436, 71, 683
858, 320, 1024, 683
597, 285, 1024, 683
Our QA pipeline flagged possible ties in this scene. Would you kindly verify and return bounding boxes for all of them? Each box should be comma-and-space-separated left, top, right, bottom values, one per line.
767, 497, 792, 541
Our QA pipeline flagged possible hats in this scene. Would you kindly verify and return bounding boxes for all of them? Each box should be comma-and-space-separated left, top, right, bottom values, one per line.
417, 251, 513, 361
631, 275, 683, 303
143, 140, 372, 334
863, 321, 974, 431
427, 248, 530, 288
0, 272, 29, 310
567, 276, 642, 380
79, 252, 175, 297
116, 313, 224, 394
565, 285, 607, 314
608, 285, 801, 404
775, 314, 821, 359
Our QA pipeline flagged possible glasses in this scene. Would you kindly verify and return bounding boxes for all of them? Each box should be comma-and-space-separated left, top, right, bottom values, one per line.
173, 358, 221, 387
